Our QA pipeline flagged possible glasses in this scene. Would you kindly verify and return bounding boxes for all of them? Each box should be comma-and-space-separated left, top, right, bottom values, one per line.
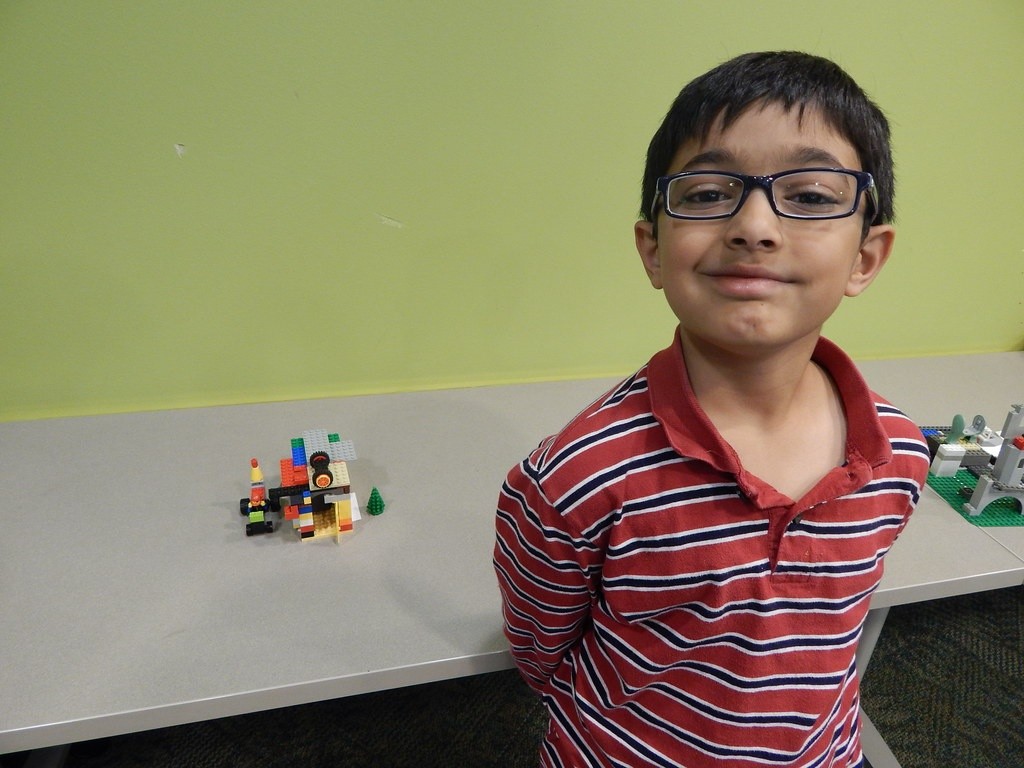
649, 170, 879, 225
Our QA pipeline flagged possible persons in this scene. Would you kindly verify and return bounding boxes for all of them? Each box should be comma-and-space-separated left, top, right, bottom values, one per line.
492, 51, 932, 768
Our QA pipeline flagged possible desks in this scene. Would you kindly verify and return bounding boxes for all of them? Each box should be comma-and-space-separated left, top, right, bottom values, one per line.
1, 358, 1024, 768
